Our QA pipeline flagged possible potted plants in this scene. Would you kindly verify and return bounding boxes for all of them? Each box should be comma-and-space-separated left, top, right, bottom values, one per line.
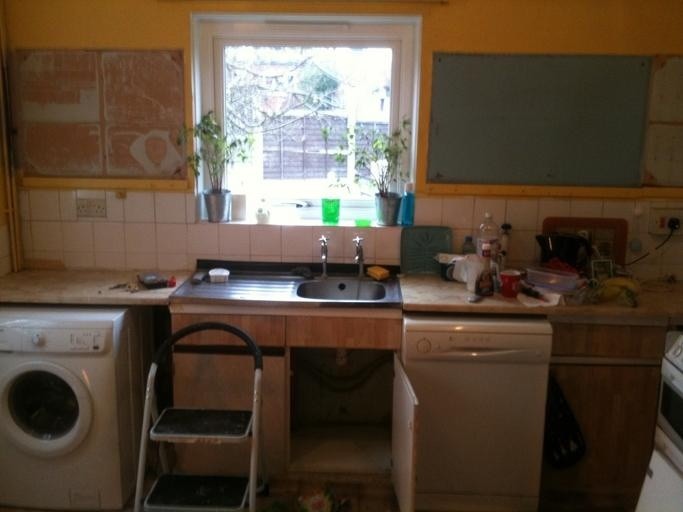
324, 116, 412, 226
176, 112, 256, 223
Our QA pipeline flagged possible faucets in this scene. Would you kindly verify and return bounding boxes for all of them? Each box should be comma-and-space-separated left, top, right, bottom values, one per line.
352, 235, 365, 278
317, 235, 329, 279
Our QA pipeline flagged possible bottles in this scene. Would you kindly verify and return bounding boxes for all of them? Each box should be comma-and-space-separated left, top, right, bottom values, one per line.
474, 212, 502, 258
462, 236, 474, 254
398, 182, 414, 225
472, 243, 494, 296
320, 171, 340, 224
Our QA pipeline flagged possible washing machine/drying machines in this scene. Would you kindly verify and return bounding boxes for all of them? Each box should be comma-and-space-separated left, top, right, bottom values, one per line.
1, 303, 154, 510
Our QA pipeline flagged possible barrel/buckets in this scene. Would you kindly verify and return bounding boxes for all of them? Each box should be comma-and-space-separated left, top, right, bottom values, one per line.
375, 192, 401, 226
202, 188, 233, 222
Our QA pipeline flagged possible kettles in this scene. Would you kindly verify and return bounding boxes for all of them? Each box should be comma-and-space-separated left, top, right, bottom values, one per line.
534, 232, 592, 268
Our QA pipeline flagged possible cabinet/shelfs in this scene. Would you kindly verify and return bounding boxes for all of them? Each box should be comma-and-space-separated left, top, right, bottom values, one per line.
540, 322, 669, 512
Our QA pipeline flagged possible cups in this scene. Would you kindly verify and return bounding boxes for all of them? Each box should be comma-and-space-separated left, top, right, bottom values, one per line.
229, 194, 247, 220
499, 269, 521, 299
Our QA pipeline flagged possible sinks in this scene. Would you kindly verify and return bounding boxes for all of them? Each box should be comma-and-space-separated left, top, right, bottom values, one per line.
293, 270, 404, 304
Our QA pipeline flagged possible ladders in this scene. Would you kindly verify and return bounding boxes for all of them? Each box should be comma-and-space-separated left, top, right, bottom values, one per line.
133, 322, 264, 512
